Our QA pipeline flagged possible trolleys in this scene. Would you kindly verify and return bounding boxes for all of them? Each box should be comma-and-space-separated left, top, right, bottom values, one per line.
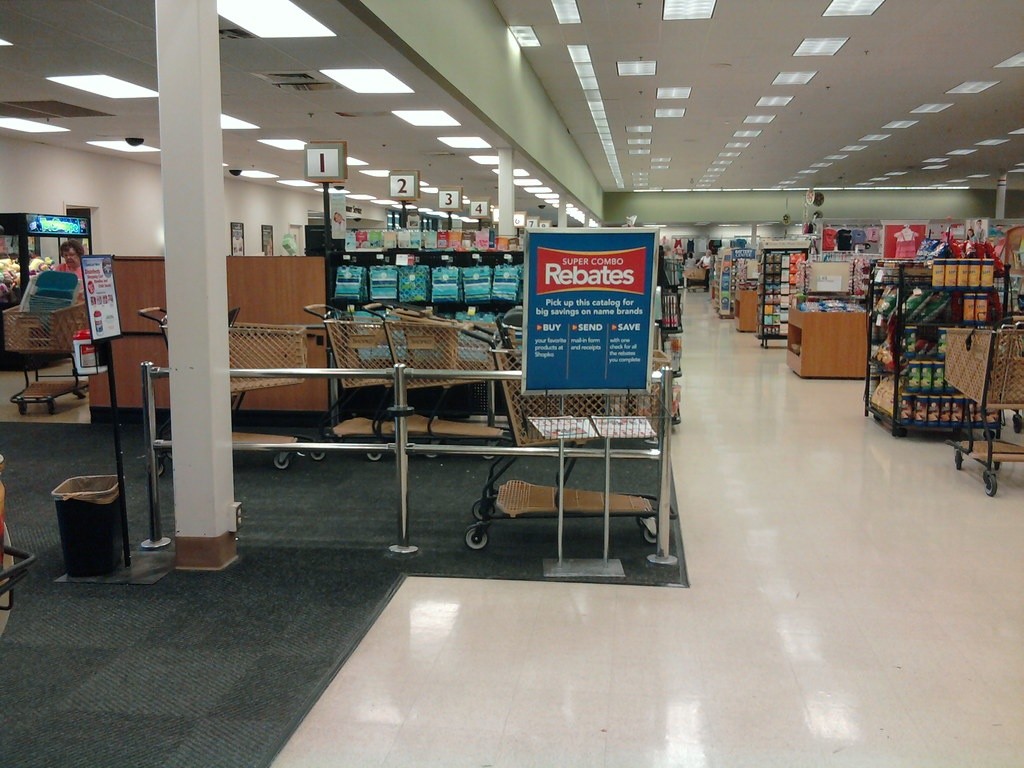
304, 302, 514, 462
943, 321, 1024, 497
2, 290, 91, 416
137, 305, 325, 476
461, 322, 682, 550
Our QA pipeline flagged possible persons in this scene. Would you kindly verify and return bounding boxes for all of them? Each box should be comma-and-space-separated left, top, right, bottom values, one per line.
688, 253, 696, 268
695, 250, 714, 292
56, 239, 88, 398
966, 220, 984, 244
676, 245, 684, 263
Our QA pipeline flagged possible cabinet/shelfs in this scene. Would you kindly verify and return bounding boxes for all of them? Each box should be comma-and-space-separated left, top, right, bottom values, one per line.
862, 260, 1009, 443
754, 239, 811, 348
787, 305, 867, 380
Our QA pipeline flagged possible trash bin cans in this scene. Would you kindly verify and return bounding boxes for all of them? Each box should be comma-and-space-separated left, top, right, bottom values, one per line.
51, 475, 126, 577
